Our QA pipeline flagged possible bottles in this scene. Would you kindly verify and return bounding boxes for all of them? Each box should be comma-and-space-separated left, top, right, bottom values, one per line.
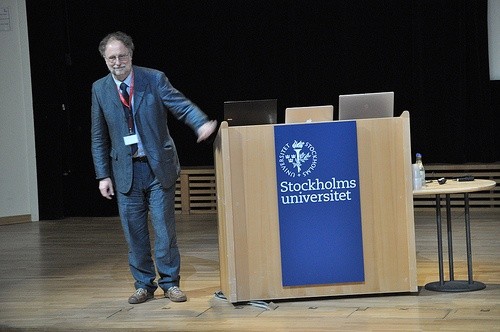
415, 153, 426, 186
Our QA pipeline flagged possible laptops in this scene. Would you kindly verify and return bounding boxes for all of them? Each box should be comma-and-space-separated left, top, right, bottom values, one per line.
224, 99, 276, 126
339, 92, 394, 120
285, 105, 334, 124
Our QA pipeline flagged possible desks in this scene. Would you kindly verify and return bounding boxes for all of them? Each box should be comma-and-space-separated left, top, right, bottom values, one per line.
413, 179, 496, 293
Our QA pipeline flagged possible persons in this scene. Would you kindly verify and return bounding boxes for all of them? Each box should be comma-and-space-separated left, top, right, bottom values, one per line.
91, 32, 217, 304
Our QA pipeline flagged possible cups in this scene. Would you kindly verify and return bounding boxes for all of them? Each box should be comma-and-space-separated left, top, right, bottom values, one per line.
412, 164, 421, 190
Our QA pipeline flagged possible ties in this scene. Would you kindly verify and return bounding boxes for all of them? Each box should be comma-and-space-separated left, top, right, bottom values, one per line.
119, 83, 139, 155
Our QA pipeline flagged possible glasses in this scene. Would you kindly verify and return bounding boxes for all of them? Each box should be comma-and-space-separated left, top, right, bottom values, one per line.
103, 50, 131, 63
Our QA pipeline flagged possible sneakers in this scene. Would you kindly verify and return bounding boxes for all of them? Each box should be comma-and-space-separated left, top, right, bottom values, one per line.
128, 287, 154, 304
164, 286, 187, 302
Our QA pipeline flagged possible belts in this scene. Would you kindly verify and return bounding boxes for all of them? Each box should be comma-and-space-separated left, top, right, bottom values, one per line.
132, 157, 149, 163
